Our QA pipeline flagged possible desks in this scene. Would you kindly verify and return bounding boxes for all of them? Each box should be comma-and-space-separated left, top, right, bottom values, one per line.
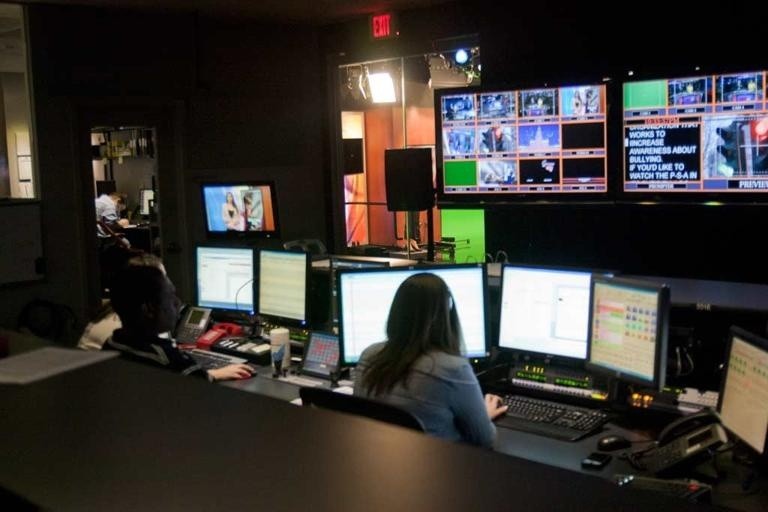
102, 312, 720, 501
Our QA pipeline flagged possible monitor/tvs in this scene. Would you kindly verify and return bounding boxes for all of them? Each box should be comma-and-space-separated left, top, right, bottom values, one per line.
616, 61, 768, 209
140, 190, 155, 217
198, 179, 279, 245
256, 246, 313, 329
434, 76, 621, 205
190, 241, 258, 318
495, 263, 617, 366
581, 272, 671, 394
712, 324, 768, 462
333, 262, 493, 368
298, 329, 342, 380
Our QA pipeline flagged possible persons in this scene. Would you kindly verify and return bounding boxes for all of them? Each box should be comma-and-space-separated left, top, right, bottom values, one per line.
224, 192, 239, 229
108, 255, 256, 386
355, 273, 509, 453
95, 182, 131, 254
79, 255, 170, 355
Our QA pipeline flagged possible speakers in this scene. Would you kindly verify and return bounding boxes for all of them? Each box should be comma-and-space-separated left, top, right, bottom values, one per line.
384, 148, 434, 212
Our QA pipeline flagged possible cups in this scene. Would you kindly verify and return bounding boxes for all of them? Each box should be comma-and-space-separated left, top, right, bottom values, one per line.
270, 329, 292, 369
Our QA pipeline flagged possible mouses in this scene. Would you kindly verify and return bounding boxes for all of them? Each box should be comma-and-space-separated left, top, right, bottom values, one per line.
249, 367, 258, 377
596, 433, 633, 453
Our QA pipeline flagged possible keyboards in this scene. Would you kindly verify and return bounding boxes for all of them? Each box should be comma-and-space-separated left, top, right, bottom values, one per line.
492, 392, 610, 443
189, 347, 248, 371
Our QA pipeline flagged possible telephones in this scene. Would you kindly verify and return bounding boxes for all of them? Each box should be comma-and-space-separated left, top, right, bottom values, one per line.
618, 410, 729, 478
161, 304, 212, 346
178, 323, 242, 349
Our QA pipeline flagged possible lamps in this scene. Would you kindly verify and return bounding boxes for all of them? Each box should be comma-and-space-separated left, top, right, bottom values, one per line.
367, 63, 397, 104
428, 57, 469, 88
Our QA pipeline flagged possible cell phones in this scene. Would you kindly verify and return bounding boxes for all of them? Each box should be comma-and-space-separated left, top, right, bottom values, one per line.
580, 449, 613, 471
